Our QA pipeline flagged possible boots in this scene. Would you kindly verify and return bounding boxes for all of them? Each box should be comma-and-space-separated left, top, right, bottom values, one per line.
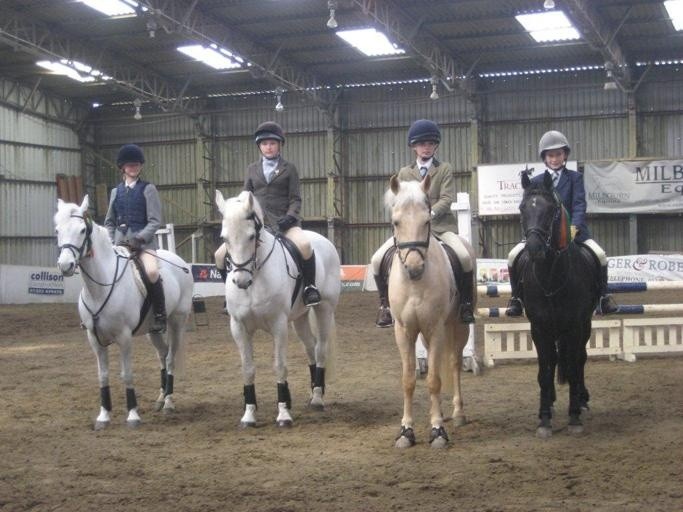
505, 266, 523, 315
301, 250, 320, 303
461, 271, 474, 321
602, 266, 617, 312
374, 274, 392, 325
151, 275, 166, 331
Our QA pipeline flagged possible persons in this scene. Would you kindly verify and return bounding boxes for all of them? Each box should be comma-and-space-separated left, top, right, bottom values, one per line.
79, 143, 167, 334
371, 120, 476, 328
214, 122, 322, 316
506, 130, 623, 316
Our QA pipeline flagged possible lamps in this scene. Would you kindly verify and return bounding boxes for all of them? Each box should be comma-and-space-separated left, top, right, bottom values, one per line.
275, 86, 286, 113
428, 74, 439, 101
325, 1, 340, 31
601, 61, 617, 92
129, 95, 144, 121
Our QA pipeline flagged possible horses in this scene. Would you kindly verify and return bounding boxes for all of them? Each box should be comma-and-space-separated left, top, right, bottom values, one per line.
215, 189, 341, 429
384, 170, 474, 451
51, 194, 194, 432
509, 169, 605, 438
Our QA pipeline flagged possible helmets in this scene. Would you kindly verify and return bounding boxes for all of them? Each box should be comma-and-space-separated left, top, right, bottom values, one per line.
117, 145, 144, 163
254, 122, 284, 143
408, 120, 440, 144
538, 130, 570, 155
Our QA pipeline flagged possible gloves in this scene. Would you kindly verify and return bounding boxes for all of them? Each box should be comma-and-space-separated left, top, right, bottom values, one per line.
277, 216, 291, 230
128, 238, 141, 252
